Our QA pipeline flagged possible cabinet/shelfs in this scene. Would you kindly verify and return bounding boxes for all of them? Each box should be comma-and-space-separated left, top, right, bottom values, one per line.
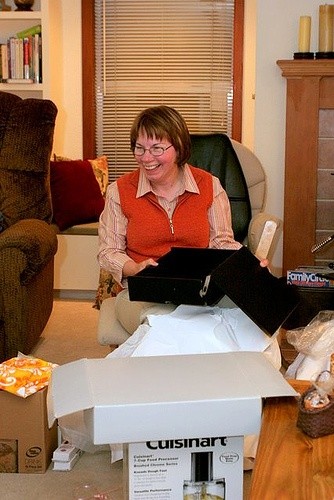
0, 0, 50, 100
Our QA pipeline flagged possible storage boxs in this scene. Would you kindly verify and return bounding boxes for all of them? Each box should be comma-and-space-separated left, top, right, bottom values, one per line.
0, 247, 334, 500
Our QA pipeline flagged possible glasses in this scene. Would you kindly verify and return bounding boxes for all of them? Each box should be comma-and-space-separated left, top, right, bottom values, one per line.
130, 144, 173, 156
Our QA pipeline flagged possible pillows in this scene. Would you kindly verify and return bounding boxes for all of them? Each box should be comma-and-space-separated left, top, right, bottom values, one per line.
48, 155, 108, 231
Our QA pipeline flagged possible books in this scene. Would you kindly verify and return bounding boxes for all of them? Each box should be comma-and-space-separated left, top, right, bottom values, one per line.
0, 33, 42, 83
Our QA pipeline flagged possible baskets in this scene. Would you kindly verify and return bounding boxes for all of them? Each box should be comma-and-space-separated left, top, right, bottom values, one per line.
287, 378, 325, 401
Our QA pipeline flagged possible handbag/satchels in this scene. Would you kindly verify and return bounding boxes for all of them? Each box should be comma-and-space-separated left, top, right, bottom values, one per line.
296, 370, 334, 439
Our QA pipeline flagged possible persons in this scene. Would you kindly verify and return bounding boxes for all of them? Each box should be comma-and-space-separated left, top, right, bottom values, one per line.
97, 106, 269, 335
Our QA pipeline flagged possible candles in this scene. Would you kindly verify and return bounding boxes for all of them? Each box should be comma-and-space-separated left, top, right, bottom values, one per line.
319, 3, 334, 52
298, 16, 311, 52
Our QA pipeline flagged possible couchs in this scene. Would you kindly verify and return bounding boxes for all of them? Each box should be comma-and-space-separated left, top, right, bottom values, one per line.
0, 92, 57, 363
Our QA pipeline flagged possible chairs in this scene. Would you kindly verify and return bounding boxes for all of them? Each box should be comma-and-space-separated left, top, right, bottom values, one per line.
95, 133, 282, 356
